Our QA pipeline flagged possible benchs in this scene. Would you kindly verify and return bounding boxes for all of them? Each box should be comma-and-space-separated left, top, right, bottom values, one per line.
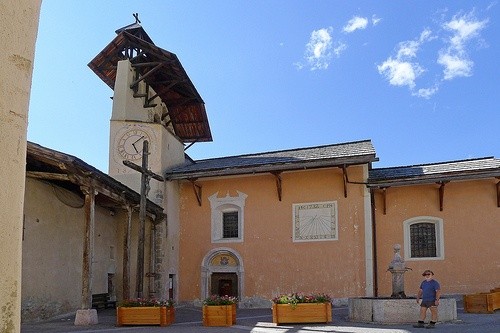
92, 293, 118, 312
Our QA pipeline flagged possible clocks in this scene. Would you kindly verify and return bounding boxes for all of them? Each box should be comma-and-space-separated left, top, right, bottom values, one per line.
119, 130, 152, 161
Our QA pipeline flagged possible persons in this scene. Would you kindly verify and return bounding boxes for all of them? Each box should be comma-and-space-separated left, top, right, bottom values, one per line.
412, 270, 441, 329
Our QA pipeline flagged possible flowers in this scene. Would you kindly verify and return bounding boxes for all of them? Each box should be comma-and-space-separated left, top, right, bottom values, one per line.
202, 295, 239, 305
117, 298, 175, 308
269, 292, 332, 310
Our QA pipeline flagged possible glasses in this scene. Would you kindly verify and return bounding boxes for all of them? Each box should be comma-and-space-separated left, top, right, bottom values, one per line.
423, 273, 430, 276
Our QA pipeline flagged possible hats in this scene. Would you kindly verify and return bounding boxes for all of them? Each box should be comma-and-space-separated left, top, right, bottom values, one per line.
422, 270, 434, 276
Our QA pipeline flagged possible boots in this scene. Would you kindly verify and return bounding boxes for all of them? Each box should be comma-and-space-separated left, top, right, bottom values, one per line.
413, 320, 426, 328
426, 320, 436, 328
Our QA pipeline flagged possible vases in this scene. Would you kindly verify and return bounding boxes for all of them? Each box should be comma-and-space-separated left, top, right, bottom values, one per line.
117, 306, 175, 327
271, 301, 332, 325
202, 305, 236, 326
464, 288, 500, 314
